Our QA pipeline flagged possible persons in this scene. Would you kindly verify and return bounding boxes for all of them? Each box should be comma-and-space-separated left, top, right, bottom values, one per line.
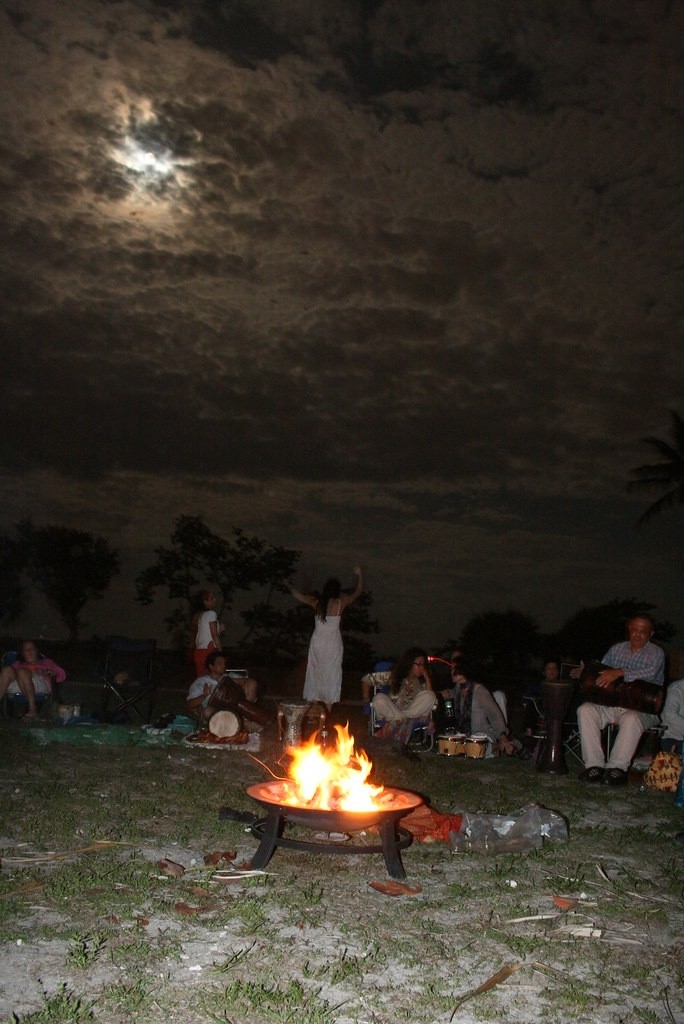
282, 565, 363, 715
360, 646, 436, 721
191, 589, 226, 680
522, 615, 684, 809
439, 665, 514, 755
0, 640, 67, 722
186, 652, 262, 733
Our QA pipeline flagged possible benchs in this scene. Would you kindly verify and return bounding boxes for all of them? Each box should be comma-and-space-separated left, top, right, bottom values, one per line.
563, 675, 664, 771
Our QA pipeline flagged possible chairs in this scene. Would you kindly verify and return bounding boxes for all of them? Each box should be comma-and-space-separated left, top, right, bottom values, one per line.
101, 636, 157, 726
367, 661, 434, 753
1, 651, 54, 720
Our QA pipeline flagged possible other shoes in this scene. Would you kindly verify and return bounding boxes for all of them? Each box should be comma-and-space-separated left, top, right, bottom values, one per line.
22, 712, 39, 724
674, 832, 684, 845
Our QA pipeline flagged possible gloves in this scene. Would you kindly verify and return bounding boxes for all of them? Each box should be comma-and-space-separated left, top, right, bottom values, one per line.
362, 703, 371, 715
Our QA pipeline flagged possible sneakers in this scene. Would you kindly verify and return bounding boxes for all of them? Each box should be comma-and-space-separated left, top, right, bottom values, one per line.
604, 767, 626, 786
578, 766, 603, 781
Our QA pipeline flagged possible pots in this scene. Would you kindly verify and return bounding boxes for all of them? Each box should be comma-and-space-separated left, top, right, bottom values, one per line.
246, 780, 430, 830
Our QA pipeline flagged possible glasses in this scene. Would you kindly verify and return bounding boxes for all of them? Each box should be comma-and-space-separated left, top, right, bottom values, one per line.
413, 661, 426, 668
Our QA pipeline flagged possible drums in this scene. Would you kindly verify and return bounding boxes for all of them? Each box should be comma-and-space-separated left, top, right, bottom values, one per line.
208, 709, 243, 738
280, 698, 312, 752
536, 682, 576, 775
578, 660, 666, 716
437, 732, 489, 759
207, 675, 275, 729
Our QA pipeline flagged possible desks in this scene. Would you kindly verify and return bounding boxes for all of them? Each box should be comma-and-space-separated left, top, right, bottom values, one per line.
56, 672, 112, 719
